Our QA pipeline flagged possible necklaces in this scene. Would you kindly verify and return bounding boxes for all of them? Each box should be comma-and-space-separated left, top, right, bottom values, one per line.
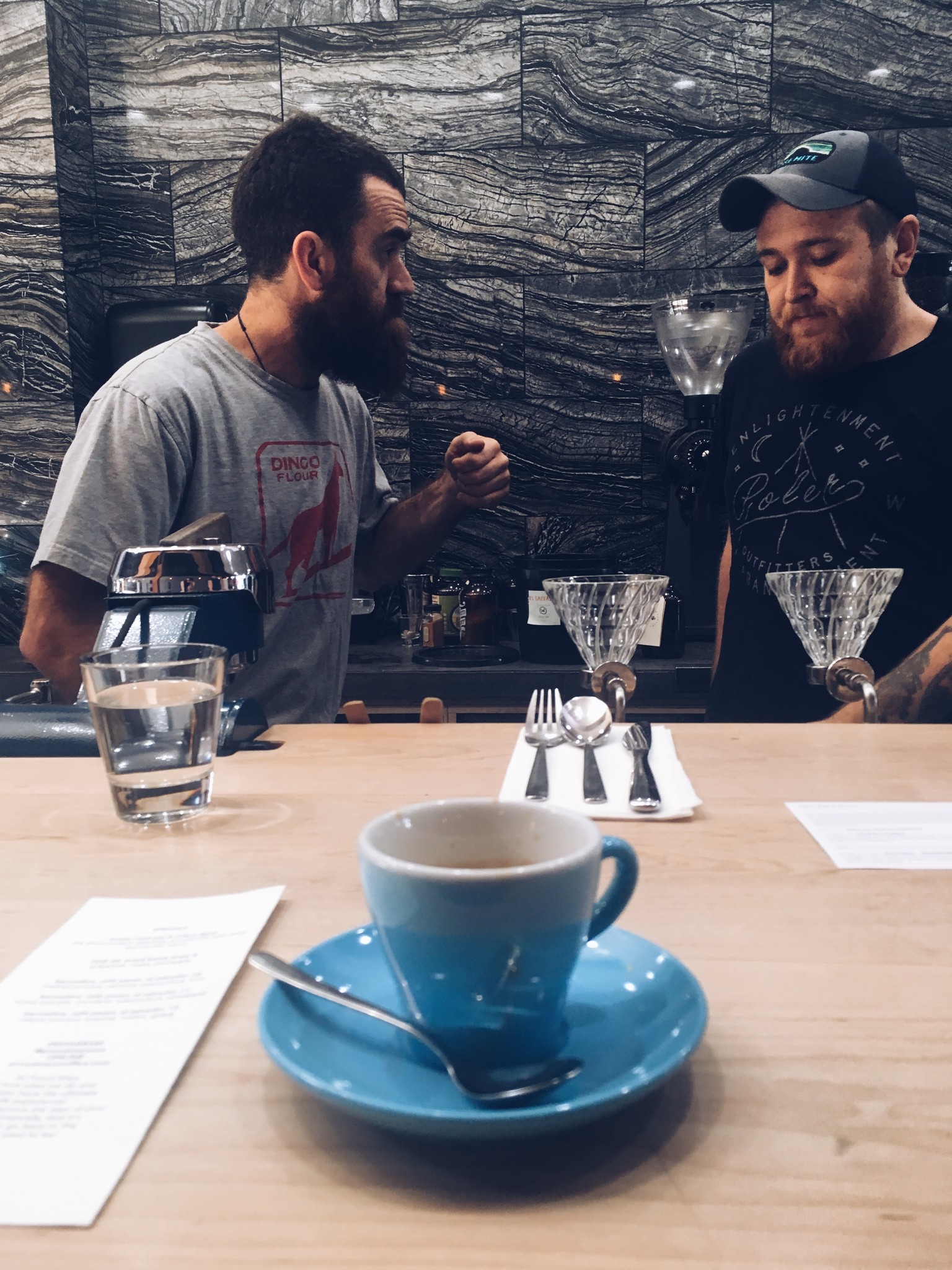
238, 310, 266, 372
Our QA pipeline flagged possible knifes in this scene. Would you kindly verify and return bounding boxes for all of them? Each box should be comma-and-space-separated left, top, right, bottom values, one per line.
621, 718, 664, 809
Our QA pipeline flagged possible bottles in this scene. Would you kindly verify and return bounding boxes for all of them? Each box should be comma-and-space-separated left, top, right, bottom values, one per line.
420, 568, 499, 649
649, 586, 686, 657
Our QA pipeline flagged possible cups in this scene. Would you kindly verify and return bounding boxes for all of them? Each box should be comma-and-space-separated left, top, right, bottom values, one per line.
506, 608, 521, 642
353, 796, 641, 1071
78, 641, 229, 818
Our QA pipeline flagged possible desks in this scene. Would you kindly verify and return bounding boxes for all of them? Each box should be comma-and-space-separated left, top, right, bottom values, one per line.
0, 720, 952, 1270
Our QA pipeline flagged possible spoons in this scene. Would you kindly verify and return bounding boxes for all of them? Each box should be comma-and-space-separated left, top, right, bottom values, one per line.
557, 695, 614, 804
249, 949, 584, 1101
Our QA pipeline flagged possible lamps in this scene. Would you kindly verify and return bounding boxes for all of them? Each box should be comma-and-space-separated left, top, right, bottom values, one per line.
652, 296, 757, 499
766, 566, 906, 723
543, 574, 669, 722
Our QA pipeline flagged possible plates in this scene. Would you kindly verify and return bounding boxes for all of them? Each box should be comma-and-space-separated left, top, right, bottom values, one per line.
259, 923, 710, 1141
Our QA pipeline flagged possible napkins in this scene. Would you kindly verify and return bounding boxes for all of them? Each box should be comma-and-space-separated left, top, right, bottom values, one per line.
499, 722, 704, 820
785, 802, 952, 871
0, 881, 287, 1226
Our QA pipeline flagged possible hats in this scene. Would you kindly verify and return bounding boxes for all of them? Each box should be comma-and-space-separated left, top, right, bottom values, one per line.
718, 129, 917, 233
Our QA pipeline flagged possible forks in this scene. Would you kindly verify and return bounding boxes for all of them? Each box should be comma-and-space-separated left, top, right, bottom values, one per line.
519, 688, 571, 802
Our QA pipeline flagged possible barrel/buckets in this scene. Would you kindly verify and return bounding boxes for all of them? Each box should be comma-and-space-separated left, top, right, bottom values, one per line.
512, 553, 616, 663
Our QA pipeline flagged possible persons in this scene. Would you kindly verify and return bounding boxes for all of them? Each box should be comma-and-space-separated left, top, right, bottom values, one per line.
702, 129, 952, 724
19, 111, 511, 724
